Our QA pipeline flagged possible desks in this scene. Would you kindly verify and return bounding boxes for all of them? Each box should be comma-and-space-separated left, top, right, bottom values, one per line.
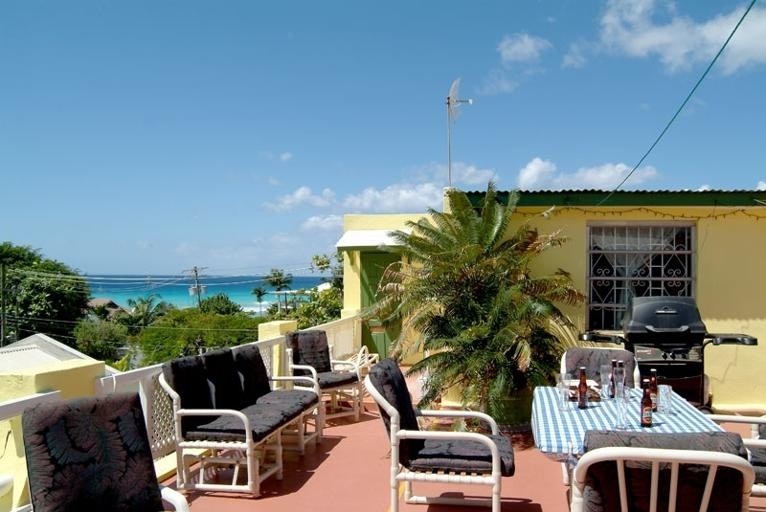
528, 384, 751, 502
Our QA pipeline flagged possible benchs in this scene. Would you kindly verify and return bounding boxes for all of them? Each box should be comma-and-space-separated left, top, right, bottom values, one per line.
159, 344, 325, 499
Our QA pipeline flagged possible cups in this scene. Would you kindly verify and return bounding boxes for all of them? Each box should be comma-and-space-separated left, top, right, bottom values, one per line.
657, 385, 672, 414
600, 365, 612, 400
613, 367, 625, 398
616, 386, 630, 429
557, 373, 571, 412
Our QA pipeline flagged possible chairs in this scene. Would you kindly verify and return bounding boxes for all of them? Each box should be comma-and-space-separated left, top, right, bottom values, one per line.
283, 329, 364, 431
18, 391, 164, 510
364, 359, 517, 511
701, 411, 765, 503
560, 347, 642, 486
567, 430, 756, 511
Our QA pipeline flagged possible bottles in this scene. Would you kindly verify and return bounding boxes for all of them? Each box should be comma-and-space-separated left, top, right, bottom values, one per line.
640, 379, 653, 427
609, 358, 617, 398
578, 366, 588, 409
649, 368, 658, 412
618, 360, 627, 386
